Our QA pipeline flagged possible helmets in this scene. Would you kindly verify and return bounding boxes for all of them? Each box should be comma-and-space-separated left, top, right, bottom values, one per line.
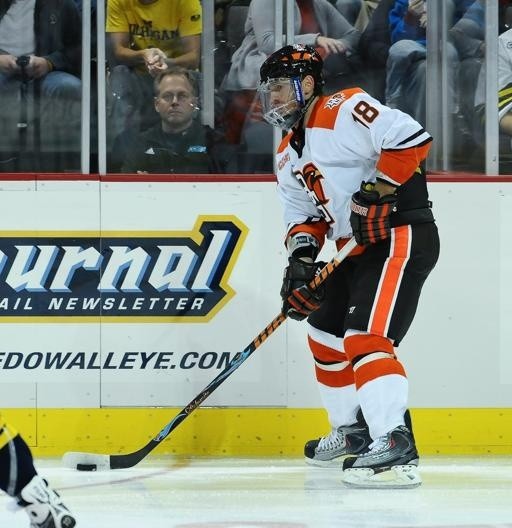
256, 43, 323, 130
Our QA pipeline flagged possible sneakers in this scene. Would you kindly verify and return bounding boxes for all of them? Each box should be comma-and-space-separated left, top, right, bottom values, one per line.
21, 479, 78, 527
304, 406, 374, 460
342, 410, 420, 475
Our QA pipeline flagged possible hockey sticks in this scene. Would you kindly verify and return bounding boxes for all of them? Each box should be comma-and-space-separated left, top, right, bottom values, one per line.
63, 236, 360, 472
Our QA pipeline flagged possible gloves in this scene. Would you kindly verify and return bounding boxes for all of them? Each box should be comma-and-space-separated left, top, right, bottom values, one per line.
280, 258, 329, 322
350, 181, 399, 247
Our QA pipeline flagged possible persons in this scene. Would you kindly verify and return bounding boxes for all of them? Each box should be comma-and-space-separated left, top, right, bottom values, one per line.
0, 415, 77, 527
1, 0, 512, 175
259, 43, 420, 474
